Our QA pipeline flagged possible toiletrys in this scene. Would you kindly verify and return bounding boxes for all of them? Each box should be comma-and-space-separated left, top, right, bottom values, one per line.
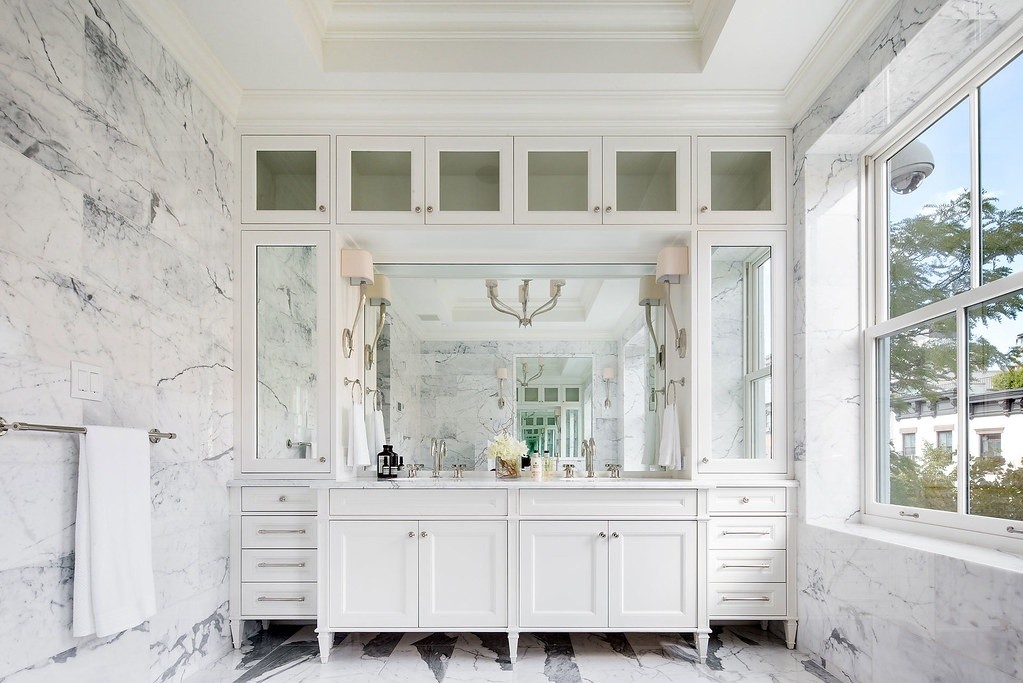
530, 450, 542, 474
377, 445, 391, 478
389, 445, 398, 478
542, 450, 554, 474
398, 455, 406, 471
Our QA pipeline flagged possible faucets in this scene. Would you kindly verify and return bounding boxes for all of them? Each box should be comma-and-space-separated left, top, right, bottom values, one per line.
586, 437, 597, 478
438, 439, 448, 471
580, 439, 589, 471
429, 437, 443, 478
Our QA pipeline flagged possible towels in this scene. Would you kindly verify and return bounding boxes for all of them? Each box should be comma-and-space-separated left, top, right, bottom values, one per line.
657, 402, 681, 471
367, 410, 387, 465
346, 400, 371, 468
72, 425, 157, 639
640, 411, 661, 465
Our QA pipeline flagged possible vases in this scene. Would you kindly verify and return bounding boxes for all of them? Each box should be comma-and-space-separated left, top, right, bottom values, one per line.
495, 456, 522, 480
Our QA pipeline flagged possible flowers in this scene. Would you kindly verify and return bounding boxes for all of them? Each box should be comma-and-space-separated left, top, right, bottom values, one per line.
487, 435, 527, 461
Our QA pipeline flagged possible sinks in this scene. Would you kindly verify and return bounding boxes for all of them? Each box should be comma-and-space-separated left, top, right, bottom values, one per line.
560, 477, 631, 482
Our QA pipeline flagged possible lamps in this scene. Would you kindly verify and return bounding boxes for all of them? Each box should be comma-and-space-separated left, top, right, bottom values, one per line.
603, 368, 614, 406
496, 368, 509, 408
516, 357, 545, 387
656, 246, 691, 360
486, 278, 567, 328
554, 407, 563, 431
341, 248, 374, 356
364, 276, 393, 369
640, 275, 664, 365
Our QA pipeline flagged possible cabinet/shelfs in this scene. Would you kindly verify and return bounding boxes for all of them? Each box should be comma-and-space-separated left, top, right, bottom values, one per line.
708, 487, 791, 617
241, 229, 334, 480
697, 136, 788, 229
513, 135, 694, 226
241, 133, 333, 226
334, 136, 516, 225
239, 485, 324, 620
693, 233, 795, 482
327, 490, 514, 631
518, 490, 699, 629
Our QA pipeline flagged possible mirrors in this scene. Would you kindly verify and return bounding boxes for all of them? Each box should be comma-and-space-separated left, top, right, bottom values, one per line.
359, 261, 672, 471
257, 246, 317, 459
711, 246, 771, 459
